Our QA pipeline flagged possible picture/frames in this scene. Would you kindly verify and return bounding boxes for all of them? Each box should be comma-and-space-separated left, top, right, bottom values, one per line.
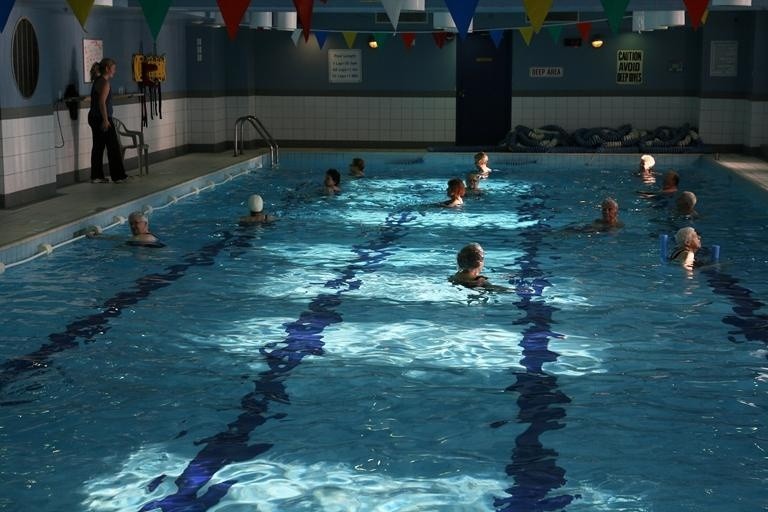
81, 35, 105, 84
708, 39, 739, 79
326, 48, 364, 85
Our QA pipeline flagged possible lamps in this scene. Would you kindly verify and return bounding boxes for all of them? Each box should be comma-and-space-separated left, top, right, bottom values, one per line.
189, 6, 297, 34
365, 34, 380, 50
631, 0, 752, 33
382, 1, 474, 34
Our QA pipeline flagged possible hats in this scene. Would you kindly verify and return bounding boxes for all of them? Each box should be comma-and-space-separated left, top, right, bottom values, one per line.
247, 193, 264, 212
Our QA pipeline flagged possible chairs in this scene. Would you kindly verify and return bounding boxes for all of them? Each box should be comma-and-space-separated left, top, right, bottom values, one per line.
109, 115, 150, 179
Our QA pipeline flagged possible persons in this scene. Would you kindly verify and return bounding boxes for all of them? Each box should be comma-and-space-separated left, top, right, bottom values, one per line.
87, 57, 139, 185
445, 242, 489, 288
635, 154, 657, 177
442, 178, 467, 208
674, 190, 697, 219
474, 152, 492, 175
669, 226, 703, 266
661, 170, 680, 193
121, 210, 160, 248
464, 174, 484, 196
348, 157, 368, 180
239, 194, 274, 223
594, 197, 624, 227
321, 170, 342, 197
